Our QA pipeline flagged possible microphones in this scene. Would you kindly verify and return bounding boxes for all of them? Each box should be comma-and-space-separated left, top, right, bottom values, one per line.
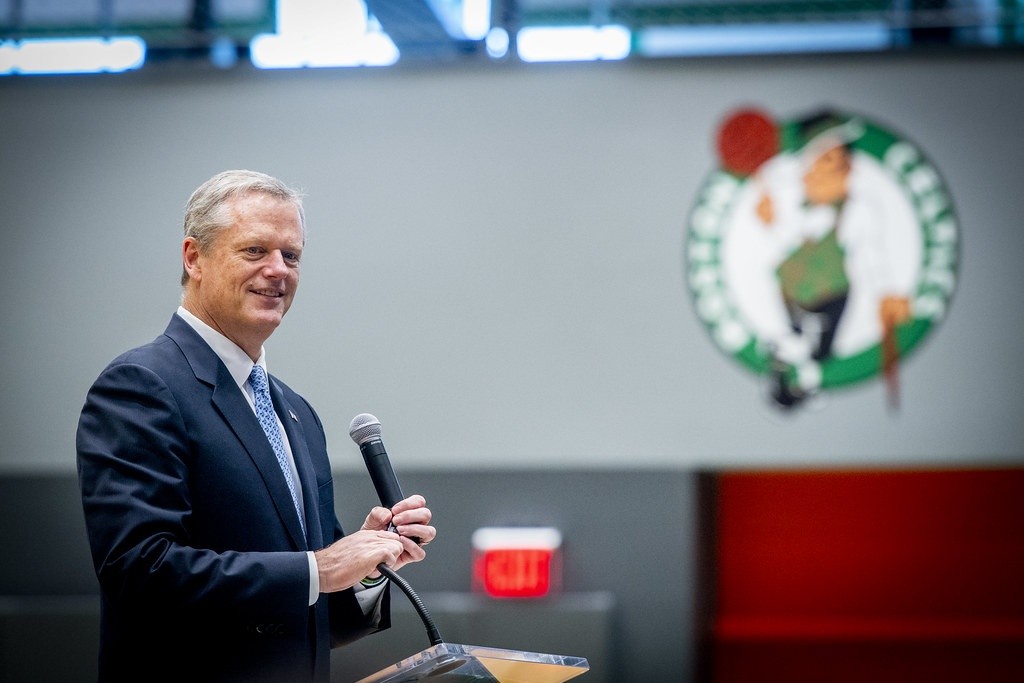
348, 413, 420, 545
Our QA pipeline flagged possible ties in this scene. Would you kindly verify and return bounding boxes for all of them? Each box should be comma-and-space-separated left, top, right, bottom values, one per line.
244, 366, 308, 550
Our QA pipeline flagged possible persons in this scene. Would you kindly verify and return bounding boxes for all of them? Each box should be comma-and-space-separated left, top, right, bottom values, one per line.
77, 171, 436, 683
